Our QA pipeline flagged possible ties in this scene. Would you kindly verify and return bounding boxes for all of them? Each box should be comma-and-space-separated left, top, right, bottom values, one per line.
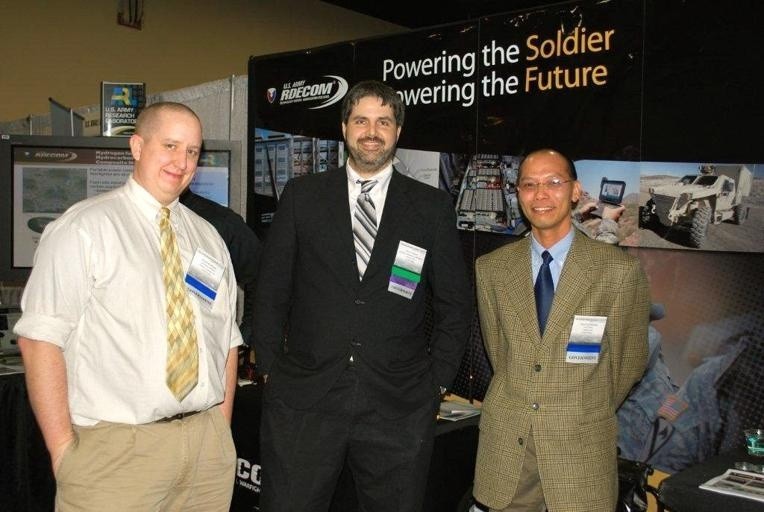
532, 249, 557, 341
352, 178, 380, 281
154, 205, 200, 404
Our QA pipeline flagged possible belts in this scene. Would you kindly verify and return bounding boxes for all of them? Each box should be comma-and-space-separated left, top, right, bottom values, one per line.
149, 410, 204, 423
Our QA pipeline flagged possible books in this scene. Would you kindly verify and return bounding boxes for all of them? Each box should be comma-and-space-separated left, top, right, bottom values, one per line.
99, 80, 146, 138
699, 468, 763, 504
438, 392, 482, 426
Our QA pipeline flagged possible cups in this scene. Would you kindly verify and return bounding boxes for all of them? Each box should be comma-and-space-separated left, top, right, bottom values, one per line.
743, 428, 763, 458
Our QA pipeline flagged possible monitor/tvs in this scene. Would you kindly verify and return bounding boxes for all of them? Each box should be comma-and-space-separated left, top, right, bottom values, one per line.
0, 134, 242, 284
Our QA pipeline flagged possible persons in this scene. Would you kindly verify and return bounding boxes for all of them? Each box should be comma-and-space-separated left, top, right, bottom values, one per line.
466, 148, 654, 511
12, 99, 249, 512
639, 310, 764, 476
615, 303, 675, 466
570, 203, 627, 247
246, 78, 474, 510
178, 184, 259, 293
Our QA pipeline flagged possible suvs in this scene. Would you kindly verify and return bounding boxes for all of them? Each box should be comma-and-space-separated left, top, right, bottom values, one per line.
639, 161, 752, 249
